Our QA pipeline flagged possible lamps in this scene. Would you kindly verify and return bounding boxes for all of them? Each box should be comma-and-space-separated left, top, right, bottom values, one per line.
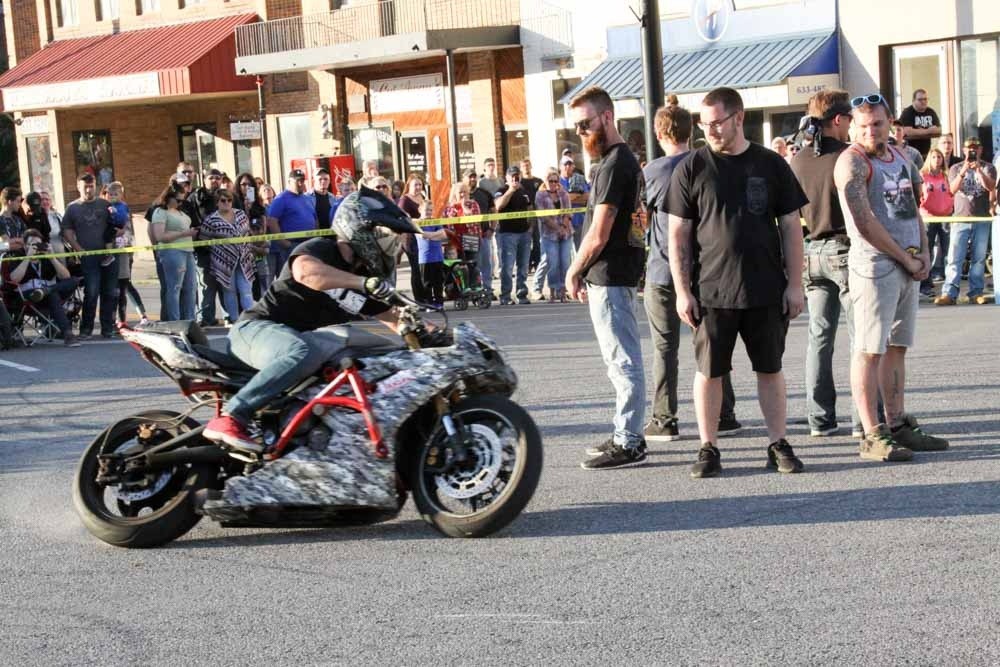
319, 102, 336, 140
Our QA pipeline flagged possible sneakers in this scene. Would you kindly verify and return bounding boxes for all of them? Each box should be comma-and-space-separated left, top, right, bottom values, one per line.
30, 287, 49, 302
202, 416, 263, 452
582, 412, 949, 478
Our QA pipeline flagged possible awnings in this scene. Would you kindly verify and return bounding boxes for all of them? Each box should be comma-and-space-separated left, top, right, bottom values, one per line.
558, 30, 840, 123
0, 13, 257, 125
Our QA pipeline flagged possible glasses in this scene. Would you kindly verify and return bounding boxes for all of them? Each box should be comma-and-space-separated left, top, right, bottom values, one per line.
833, 112, 853, 121
374, 184, 387, 189
851, 94, 890, 112
697, 112, 736, 130
549, 180, 559, 184
182, 169, 252, 204
573, 113, 604, 130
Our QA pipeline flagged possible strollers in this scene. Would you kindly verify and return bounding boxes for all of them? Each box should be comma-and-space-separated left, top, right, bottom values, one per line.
439, 227, 496, 312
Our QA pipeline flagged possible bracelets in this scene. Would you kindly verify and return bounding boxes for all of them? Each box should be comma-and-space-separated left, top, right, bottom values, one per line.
960, 174, 964, 178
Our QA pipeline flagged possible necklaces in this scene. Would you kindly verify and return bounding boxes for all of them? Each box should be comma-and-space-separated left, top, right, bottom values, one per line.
547, 190, 556, 193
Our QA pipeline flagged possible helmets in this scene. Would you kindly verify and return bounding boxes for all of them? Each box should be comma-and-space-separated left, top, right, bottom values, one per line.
331, 189, 424, 278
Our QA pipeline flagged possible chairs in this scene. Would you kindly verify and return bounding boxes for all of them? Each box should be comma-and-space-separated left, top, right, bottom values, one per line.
0, 252, 87, 348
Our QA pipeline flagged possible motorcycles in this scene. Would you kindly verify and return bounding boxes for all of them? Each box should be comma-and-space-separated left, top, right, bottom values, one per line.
68, 274, 545, 551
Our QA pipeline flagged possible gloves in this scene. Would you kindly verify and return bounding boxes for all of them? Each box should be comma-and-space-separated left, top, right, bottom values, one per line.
360, 276, 394, 301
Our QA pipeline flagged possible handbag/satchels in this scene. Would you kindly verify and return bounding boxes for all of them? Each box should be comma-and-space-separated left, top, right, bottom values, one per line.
462, 234, 479, 251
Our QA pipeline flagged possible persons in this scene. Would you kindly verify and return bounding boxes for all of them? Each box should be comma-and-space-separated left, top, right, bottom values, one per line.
101, 182, 129, 266
790, 89, 863, 438
566, 86, 651, 472
202, 188, 443, 453
640, 93, 743, 442
891, 88, 999, 308
61, 172, 120, 340
143, 162, 400, 330
444, 148, 590, 306
398, 173, 448, 311
0, 188, 81, 348
99, 185, 147, 330
660, 86, 811, 475
834, 95, 947, 464
772, 136, 800, 166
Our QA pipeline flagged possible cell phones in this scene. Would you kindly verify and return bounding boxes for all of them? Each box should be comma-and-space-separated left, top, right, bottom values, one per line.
515, 176, 520, 183
32, 242, 49, 251
247, 187, 255, 202
969, 151, 977, 162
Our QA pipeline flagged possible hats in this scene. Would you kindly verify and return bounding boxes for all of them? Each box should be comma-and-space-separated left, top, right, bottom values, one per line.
560, 148, 575, 164
464, 169, 476, 177
507, 166, 520, 176
289, 167, 330, 177
964, 136, 982, 148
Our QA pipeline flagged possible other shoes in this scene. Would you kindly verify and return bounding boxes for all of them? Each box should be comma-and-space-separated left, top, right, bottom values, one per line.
920, 286, 988, 304
101, 255, 116, 266
64, 320, 155, 347
489, 294, 570, 305
